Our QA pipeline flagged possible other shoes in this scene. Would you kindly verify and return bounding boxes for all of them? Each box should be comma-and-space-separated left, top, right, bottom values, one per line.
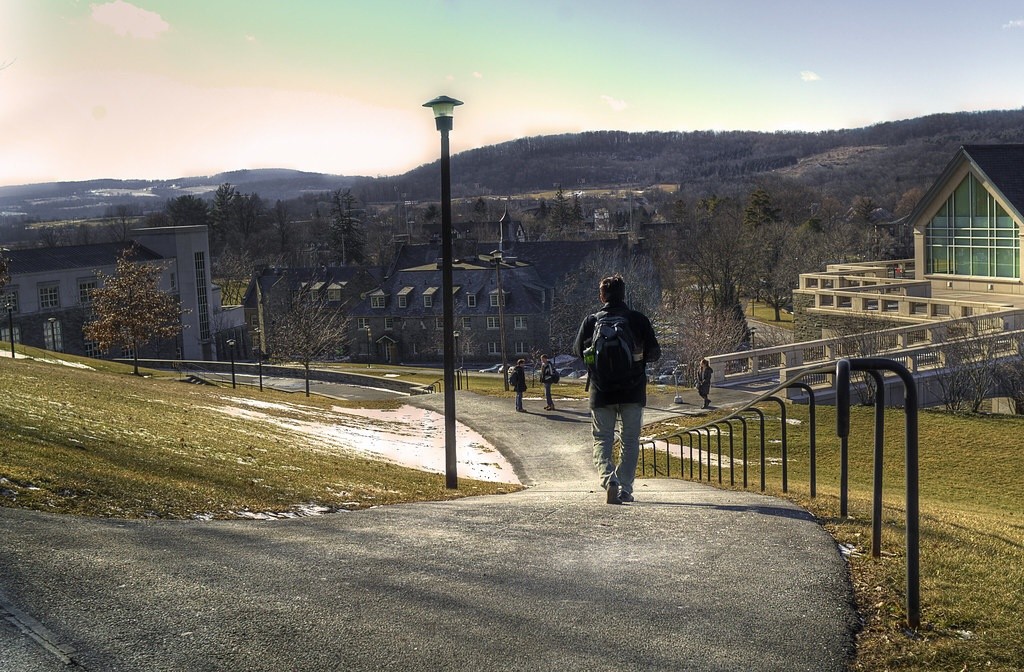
617, 490, 635, 502
544, 405, 555, 409
606, 482, 623, 505
546, 404, 557, 410
515, 409, 528, 412
701, 400, 713, 409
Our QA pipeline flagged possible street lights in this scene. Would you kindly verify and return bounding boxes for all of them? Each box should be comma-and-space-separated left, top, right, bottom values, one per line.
423, 94, 465, 490
749, 327, 756, 350
490, 248, 511, 390
5, 302, 18, 360
226, 339, 237, 390
452, 330, 461, 392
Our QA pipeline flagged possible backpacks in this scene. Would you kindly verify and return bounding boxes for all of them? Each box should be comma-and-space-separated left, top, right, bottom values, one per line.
585, 311, 645, 392
508, 368, 518, 386
550, 363, 561, 385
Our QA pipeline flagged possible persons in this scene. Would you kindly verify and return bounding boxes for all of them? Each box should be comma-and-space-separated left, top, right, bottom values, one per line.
696, 359, 713, 410
539, 355, 555, 411
513, 359, 528, 413
573, 277, 662, 505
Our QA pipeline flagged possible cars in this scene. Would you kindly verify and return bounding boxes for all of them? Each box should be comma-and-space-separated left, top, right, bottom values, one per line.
478, 358, 694, 387
